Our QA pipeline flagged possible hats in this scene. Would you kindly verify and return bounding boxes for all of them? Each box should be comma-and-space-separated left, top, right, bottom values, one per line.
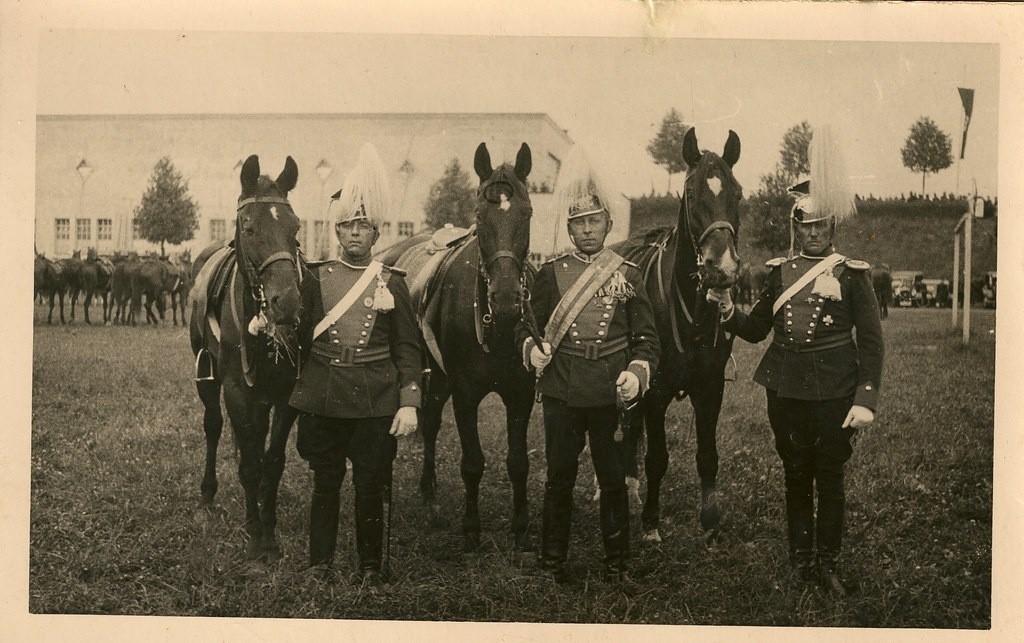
337, 184, 374, 223
568, 180, 605, 219
788, 181, 835, 223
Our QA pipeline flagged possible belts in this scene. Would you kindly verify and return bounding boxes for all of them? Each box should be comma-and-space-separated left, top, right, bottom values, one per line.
772, 331, 853, 353
556, 336, 628, 360
311, 341, 390, 363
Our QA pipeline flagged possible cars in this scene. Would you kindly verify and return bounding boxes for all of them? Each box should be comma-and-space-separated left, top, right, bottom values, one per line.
888, 271, 951, 308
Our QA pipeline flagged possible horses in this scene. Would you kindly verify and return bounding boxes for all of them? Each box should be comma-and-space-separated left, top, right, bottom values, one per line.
188, 154, 311, 564
34, 246, 192, 328
380, 141, 543, 569
606, 128, 743, 546
867, 269, 892, 319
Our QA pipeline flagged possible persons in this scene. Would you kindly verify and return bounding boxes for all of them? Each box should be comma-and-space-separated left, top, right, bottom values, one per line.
288, 144, 423, 600
705, 122, 886, 601
514, 143, 662, 598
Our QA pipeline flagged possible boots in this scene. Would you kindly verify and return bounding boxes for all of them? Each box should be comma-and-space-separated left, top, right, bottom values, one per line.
815, 479, 846, 592
542, 490, 573, 584
777, 485, 814, 592
357, 484, 383, 589
600, 490, 629, 585
309, 492, 340, 570
